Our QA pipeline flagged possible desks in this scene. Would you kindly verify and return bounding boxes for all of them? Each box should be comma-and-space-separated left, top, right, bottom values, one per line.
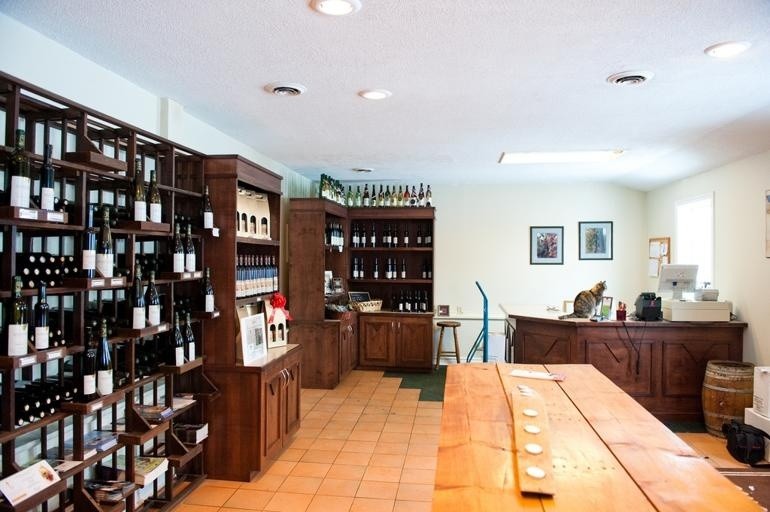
432, 361, 757, 512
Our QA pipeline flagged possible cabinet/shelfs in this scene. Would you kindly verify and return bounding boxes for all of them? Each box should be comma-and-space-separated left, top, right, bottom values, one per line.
347, 204, 437, 374
286, 198, 356, 390
168, 155, 300, 481
0, 73, 205, 512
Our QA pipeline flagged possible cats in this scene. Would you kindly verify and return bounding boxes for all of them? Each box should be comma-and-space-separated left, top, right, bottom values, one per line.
558, 280, 608, 320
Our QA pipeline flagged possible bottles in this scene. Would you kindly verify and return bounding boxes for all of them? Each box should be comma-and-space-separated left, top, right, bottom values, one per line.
4, 129, 31, 209
39, 143, 55, 211
424, 224, 428, 247
363, 183, 370, 207
96, 323, 115, 399
0, 250, 79, 289
80, 205, 96, 279
174, 215, 194, 234
113, 267, 134, 283
397, 185, 404, 208
400, 256, 406, 279
356, 223, 360, 248
391, 185, 397, 208
33, 282, 50, 352
319, 173, 346, 206
166, 311, 185, 368
372, 257, 379, 279
391, 288, 396, 312
419, 183, 425, 207
270, 324, 273, 343
385, 257, 393, 279
273, 323, 282, 342
183, 223, 196, 273
428, 255, 432, 279
170, 226, 185, 274
386, 223, 392, 248
403, 185, 410, 208
398, 288, 429, 313
237, 213, 239, 231
118, 252, 159, 280
74, 326, 98, 405
360, 257, 365, 278
377, 184, 384, 208
371, 184, 376, 207
393, 256, 398, 279
392, 224, 399, 248
352, 255, 359, 278
416, 223, 422, 247
49, 308, 119, 343
385, 185, 391, 208
204, 267, 215, 313
30, 195, 69, 214
86, 202, 129, 229
351, 224, 357, 248
427, 223, 433, 247
147, 170, 162, 224
370, 222, 376, 249
426, 185, 432, 207
28, 329, 66, 351
421, 256, 427, 279
0, 379, 79, 427
325, 222, 345, 246
3, 275, 29, 358
181, 313, 196, 364
261, 218, 267, 235
64, 343, 159, 387
241, 213, 247, 232
87, 137, 104, 154
355, 186, 361, 207
236, 253, 279, 300
157, 292, 195, 323
250, 216, 256, 234
346, 185, 354, 207
127, 266, 146, 329
95, 207, 114, 278
403, 224, 409, 247
382, 224, 386, 248
411, 186, 417, 208
360, 223, 367, 248
200, 184, 214, 230
130, 158, 147, 222
145, 271, 161, 328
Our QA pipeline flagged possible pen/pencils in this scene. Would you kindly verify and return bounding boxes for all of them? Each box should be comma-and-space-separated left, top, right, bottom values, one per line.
616, 302, 627, 312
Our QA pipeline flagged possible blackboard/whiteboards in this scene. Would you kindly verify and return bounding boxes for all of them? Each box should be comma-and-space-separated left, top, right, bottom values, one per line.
349, 292, 372, 302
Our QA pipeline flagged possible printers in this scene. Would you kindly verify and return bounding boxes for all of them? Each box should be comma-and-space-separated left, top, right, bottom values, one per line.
634, 291, 663, 322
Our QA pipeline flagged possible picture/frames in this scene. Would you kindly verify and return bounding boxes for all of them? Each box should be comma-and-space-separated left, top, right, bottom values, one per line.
577, 221, 613, 260
529, 225, 565, 266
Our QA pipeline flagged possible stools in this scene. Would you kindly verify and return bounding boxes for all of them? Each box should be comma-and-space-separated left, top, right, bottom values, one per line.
436, 321, 460, 368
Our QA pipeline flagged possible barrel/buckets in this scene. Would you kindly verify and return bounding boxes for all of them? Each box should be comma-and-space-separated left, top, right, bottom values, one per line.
702, 360, 755, 441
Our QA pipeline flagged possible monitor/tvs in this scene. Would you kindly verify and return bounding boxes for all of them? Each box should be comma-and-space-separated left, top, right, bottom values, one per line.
657, 264, 699, 300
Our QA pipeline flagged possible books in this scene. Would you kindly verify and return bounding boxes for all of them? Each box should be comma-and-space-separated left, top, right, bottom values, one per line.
84, 478, 135, 502
38, 446, 97, 461
155, 396, 196, 409
173, 422, 209, 445
134, 403, 173, 421
240, 313, 267, 366
102, 454, 168, 485
64, 434, 117, 452
21, 458, 83, 472
